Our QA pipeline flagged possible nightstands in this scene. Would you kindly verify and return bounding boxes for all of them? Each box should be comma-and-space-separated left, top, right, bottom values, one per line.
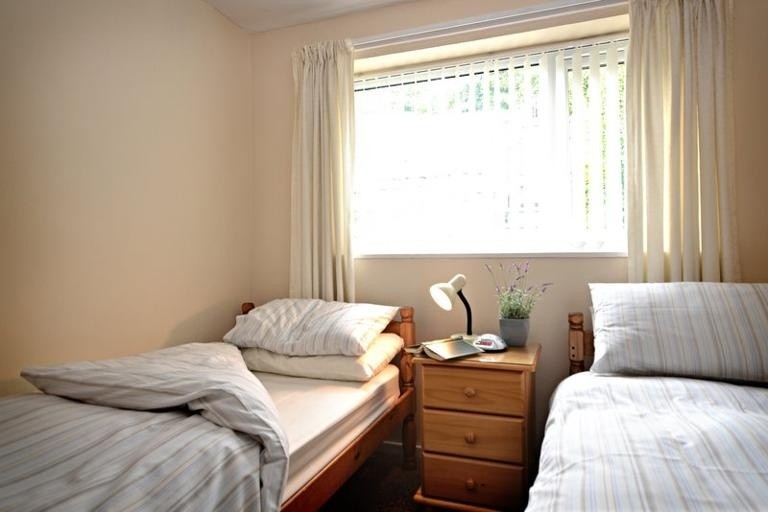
408, 339, 543, 512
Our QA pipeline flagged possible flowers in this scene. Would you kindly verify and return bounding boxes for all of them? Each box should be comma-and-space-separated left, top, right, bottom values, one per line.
482, 260, 556, 322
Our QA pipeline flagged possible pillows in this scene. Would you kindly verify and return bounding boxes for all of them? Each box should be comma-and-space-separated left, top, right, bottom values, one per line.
215, 293, 404, 385
586, 274, 768, 385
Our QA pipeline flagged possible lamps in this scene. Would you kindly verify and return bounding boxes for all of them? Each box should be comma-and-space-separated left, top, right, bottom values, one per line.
428, 274, 485, 354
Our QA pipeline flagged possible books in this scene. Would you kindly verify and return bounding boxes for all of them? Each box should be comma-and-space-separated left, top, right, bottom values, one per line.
402, 336, 486, 361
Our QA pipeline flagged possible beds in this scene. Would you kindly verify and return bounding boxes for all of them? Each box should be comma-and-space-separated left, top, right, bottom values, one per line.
524, 310, 768, 511
0, 299, 418, 510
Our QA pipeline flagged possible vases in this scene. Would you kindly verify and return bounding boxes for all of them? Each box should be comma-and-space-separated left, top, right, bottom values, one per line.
498, 317, 529, 347
